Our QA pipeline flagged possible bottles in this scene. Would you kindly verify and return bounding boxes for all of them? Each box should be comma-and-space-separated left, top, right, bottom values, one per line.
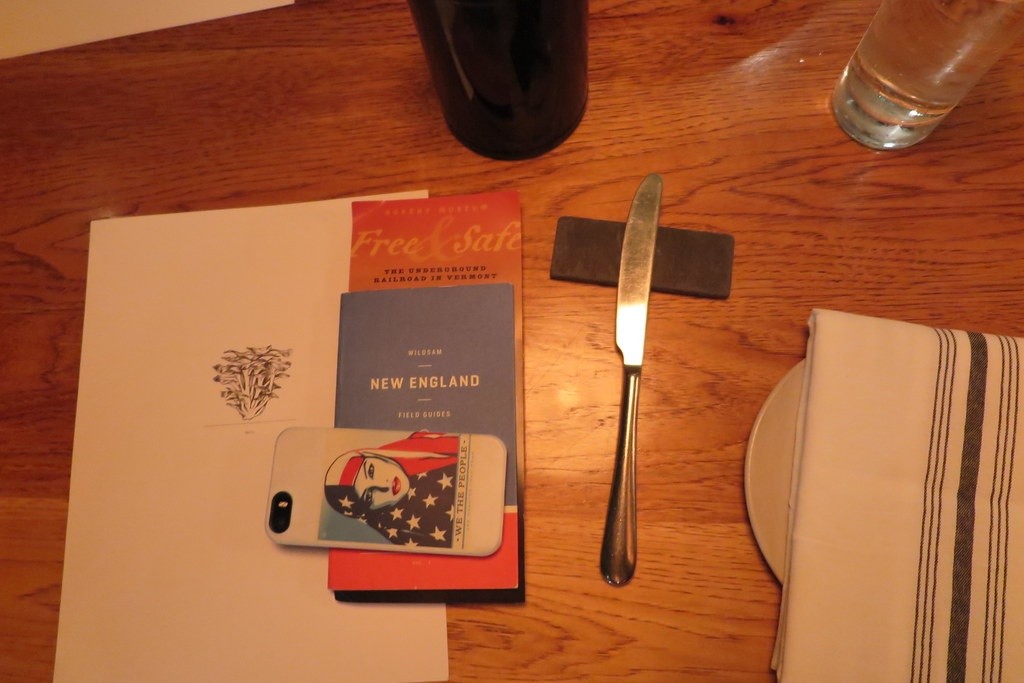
405, 0, 590, 162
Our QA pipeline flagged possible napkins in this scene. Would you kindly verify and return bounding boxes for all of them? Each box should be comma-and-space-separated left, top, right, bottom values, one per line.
770, 309, 1024, 683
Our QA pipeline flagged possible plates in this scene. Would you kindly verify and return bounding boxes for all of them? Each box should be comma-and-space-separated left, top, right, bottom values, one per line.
745, 357, 806, 586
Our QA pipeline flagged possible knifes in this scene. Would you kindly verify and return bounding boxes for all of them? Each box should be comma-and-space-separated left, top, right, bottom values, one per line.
599, 171, 663, 585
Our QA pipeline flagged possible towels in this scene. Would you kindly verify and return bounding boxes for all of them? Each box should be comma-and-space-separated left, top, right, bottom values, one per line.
767, 305, 1023, 683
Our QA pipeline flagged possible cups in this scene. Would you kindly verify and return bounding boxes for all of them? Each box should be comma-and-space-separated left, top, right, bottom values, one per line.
830, 0, 1024, 152
